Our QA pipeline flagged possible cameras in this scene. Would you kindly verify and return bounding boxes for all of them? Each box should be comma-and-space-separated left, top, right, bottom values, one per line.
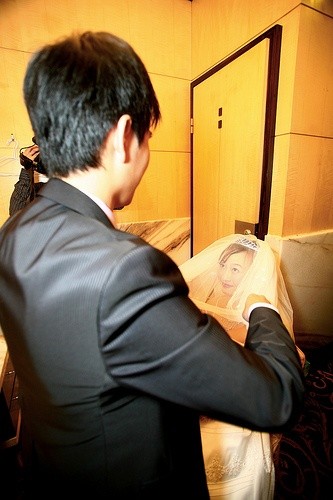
19, 136, 48, 177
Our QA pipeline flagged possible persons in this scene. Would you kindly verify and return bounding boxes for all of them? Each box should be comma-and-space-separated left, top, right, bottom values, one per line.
0, 28, 306, 500
7, 136, 53, 223
164, 231, 297, 500
